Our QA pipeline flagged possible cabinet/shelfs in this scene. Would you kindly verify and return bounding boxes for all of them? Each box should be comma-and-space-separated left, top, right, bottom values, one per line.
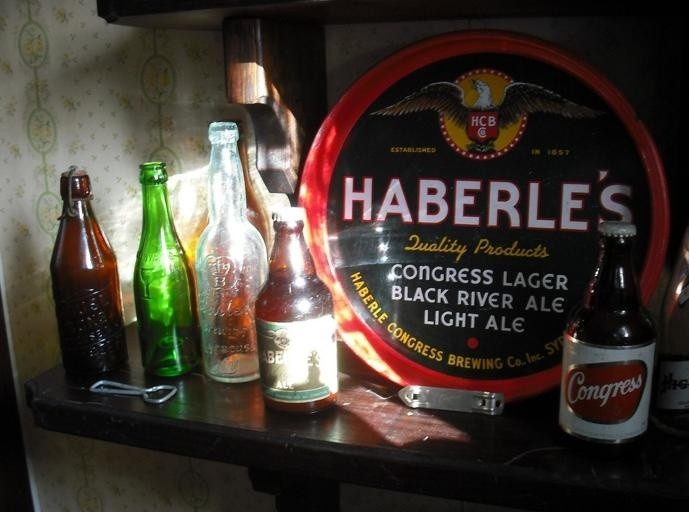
24, 0, 689, 512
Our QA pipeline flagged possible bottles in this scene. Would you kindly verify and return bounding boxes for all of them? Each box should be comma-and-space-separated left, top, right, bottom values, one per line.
253, 206, 339, 416
48, 169, 130, 375
559, 218, 658, 466
194, 121, 272, 385
654, 224, 689, 431
133, 159, 200, 382
